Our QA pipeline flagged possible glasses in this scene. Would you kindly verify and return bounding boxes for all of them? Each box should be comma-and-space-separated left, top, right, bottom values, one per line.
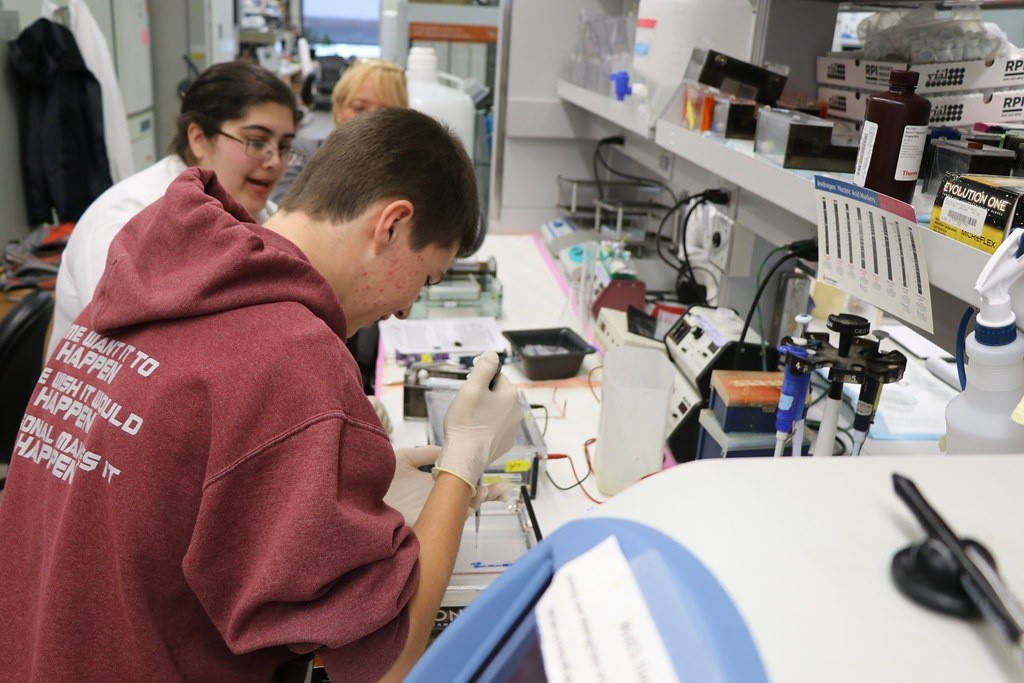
212, 127, 307, 170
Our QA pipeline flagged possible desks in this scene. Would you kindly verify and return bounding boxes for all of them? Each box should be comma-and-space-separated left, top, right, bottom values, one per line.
332, 225, 952, 615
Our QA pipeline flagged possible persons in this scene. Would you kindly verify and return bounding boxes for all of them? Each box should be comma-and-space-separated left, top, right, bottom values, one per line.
44, 62, 308, 378
330, 58, 409, 128
0, 105, 522, 683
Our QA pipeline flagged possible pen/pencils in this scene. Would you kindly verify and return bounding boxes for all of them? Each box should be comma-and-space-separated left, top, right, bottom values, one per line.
889, 470, 1024, 658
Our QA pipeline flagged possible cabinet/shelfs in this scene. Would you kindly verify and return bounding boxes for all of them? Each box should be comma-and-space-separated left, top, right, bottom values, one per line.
554, 0, 1024, 335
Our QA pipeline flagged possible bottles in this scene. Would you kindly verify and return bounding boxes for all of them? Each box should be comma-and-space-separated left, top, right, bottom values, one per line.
855, 70, 932, 205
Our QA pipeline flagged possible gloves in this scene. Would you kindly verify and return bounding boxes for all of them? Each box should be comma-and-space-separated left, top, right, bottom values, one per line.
384, 445, 509, 531
433, 349, 518, 499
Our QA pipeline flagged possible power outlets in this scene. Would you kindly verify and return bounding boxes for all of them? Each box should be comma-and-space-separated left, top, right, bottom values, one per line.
715, 176, 741, 220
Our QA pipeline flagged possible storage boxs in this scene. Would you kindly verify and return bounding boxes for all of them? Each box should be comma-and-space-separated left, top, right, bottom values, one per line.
710, 368, 810, 432
423, 389, 548, 501
697, 409, 814, 461
809, 369, 946, 457
